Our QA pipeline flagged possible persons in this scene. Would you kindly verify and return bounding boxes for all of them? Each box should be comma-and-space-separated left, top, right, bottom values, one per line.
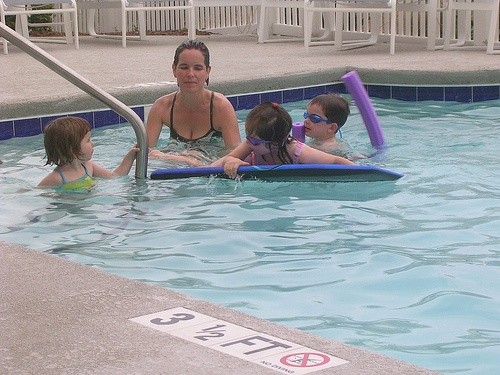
145, 39, 243, 168
302, 93, 369, 163
224, 100, 359, 180
36, 116, 141, 191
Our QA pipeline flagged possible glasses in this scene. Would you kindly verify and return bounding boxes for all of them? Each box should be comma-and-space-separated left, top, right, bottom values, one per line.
303, 112, 342, 138
246, 136, 273, 146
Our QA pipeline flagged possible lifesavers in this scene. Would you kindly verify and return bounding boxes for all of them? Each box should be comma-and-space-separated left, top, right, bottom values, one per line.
290, 70, 387, 155
